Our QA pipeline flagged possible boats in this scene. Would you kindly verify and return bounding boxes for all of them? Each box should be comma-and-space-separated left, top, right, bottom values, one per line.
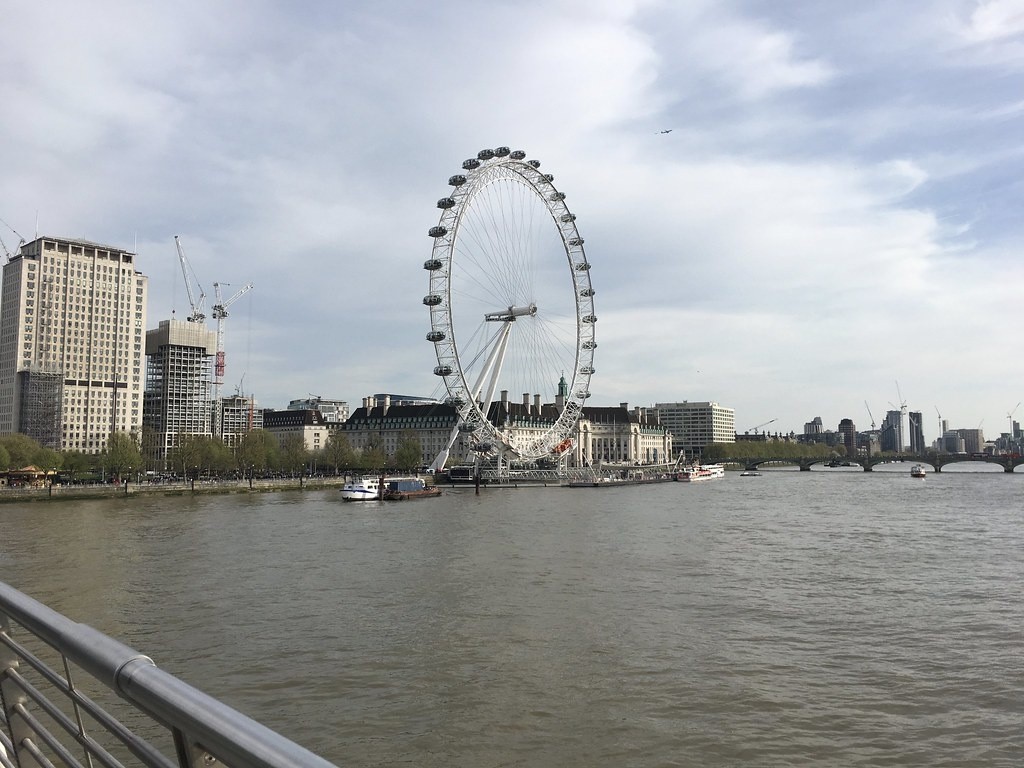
676, 465, 725, 482
910, 464, 926, 478
740, 471, 763, 476
339, 478, 442, 502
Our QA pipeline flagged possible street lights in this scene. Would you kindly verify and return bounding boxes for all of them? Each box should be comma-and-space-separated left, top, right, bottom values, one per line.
416, 461, 418, 472
128, 467, 131, 480
194, 465, 197, 480
383, 462, 386, 474
302, 463, 305, 477
251, 465, 254, 479
53, 467, 57, 485
345, 463, 348, 477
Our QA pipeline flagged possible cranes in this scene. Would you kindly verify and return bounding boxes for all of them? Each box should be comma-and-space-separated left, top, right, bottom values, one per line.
0, 219, 26, 263
888, 380, 907, 416
234, 372, 246, 398
173, 234, 206, 324
934, 405, 942, 438
749, 418, 778, 435
211, 279, 255, 442
865, 399, 877, 433
1006, 402, 1022, 437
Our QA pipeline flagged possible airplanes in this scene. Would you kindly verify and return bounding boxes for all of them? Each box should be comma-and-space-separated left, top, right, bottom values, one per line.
660, 129, 672, 134
